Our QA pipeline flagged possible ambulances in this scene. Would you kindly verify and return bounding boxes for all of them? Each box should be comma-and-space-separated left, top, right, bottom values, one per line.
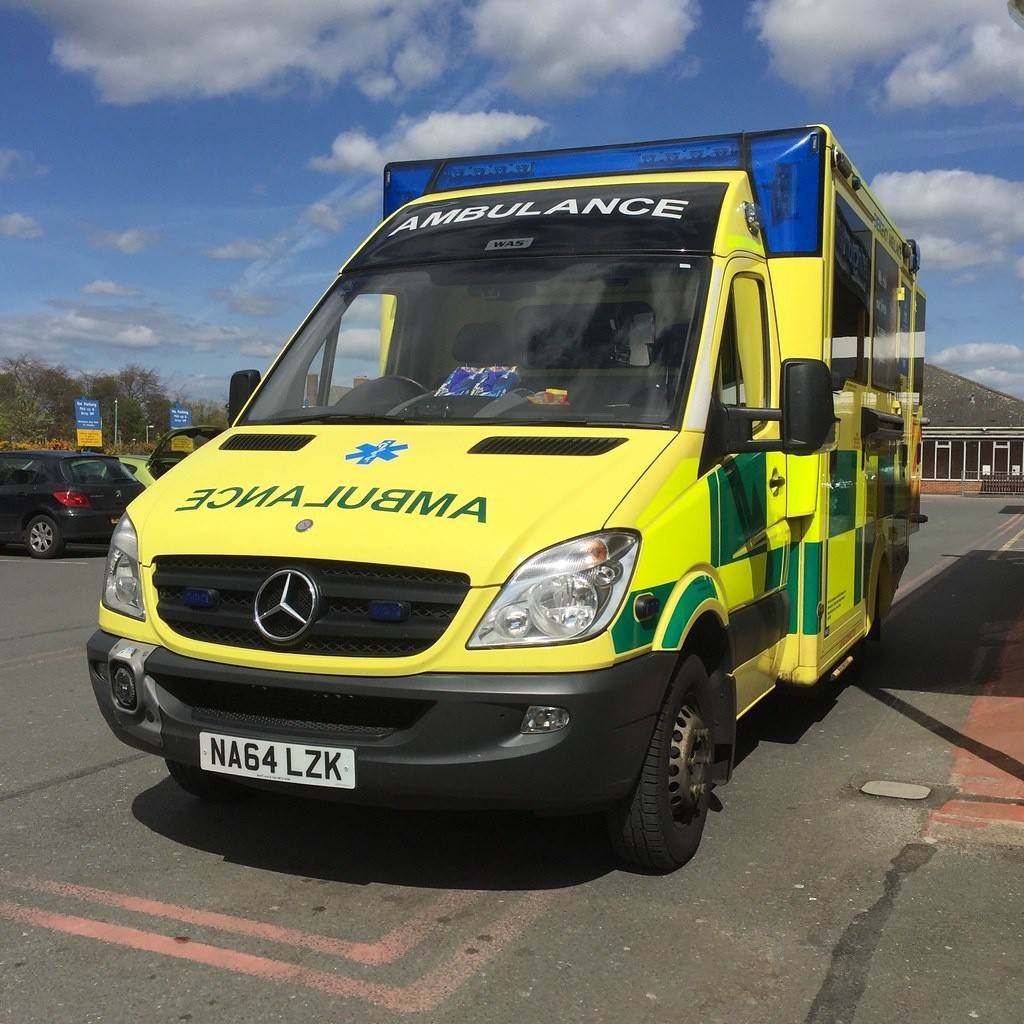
84, 119, 934, 876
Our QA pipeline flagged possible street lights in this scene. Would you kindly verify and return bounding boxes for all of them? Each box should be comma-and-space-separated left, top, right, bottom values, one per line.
145, 425, 155, 444
114, 397, 118, 446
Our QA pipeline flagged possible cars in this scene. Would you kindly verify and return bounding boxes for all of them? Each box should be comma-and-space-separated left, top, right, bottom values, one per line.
0, 450, 148, 558
70, 454, 157, 488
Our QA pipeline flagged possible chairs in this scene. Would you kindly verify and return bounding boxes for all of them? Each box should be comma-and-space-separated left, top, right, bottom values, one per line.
10, 470, 33, 483
432, 323, 507, 390
104, 468, 127, 480
627, 324, 694, 409
